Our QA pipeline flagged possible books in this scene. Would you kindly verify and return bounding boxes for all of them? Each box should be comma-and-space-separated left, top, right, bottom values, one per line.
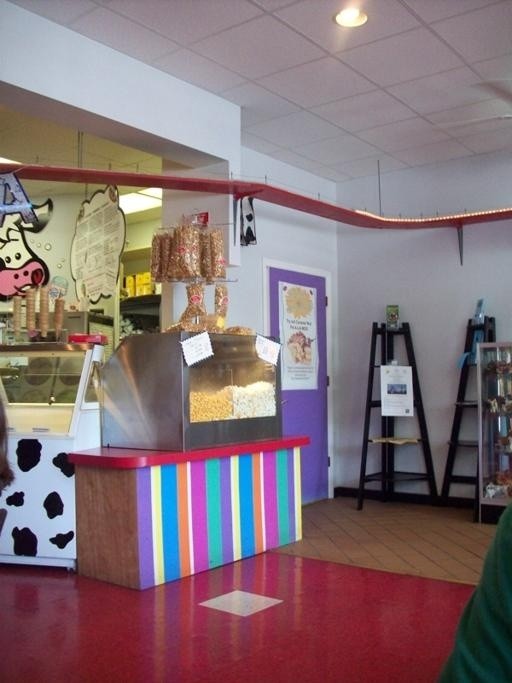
372, 435, 418, 445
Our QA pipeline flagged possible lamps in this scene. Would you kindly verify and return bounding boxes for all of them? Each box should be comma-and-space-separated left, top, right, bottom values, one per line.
334, 8, 369, 27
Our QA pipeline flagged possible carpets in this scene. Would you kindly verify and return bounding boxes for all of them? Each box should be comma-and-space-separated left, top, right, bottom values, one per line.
0, 548, 479, 683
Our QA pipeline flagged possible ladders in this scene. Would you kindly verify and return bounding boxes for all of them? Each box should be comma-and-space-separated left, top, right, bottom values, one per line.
440, 316, 501, 506
356, 321, 439, 511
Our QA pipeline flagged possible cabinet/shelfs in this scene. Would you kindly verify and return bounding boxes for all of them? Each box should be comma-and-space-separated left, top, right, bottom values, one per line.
476, 342, 512, 525
113, 206, 162, 356
0, 343, 106, 574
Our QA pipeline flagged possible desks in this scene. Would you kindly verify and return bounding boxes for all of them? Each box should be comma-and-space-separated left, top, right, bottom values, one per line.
69, 436, 312, 591
